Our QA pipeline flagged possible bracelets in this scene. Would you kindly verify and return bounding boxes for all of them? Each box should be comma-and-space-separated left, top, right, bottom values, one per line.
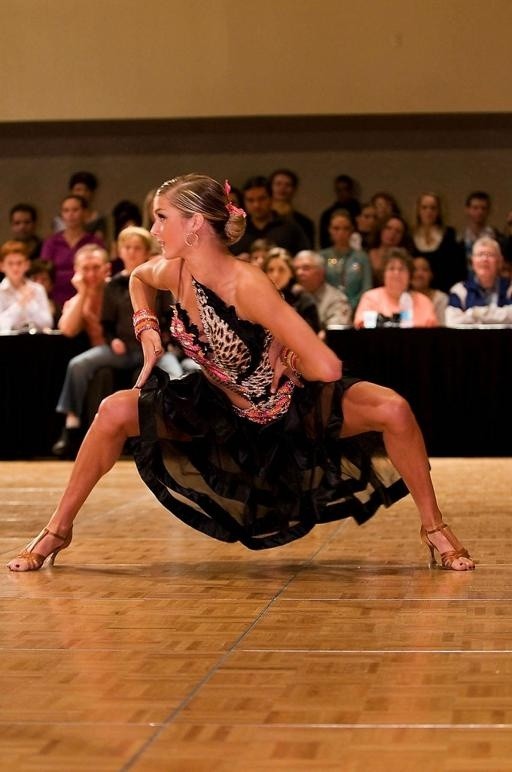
279, 345, 304, 379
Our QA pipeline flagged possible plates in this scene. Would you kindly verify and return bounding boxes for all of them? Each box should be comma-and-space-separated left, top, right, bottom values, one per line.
326, 324, 353, 331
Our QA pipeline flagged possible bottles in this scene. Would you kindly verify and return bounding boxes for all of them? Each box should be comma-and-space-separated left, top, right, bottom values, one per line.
398, 287, 414, 329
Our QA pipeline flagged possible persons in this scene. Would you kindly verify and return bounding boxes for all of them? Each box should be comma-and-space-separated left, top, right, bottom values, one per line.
7, 172, 475, 572
224, 169, 512, 329
44, 226, 183, 459
0, 169, 161, 342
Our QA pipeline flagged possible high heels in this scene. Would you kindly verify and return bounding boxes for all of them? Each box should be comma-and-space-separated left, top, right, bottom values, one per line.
7, 525, 73, 571
420, 524, 475, 571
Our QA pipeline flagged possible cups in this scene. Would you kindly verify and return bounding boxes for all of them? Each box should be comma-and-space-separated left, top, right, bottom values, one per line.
361, 309, 379, 329
